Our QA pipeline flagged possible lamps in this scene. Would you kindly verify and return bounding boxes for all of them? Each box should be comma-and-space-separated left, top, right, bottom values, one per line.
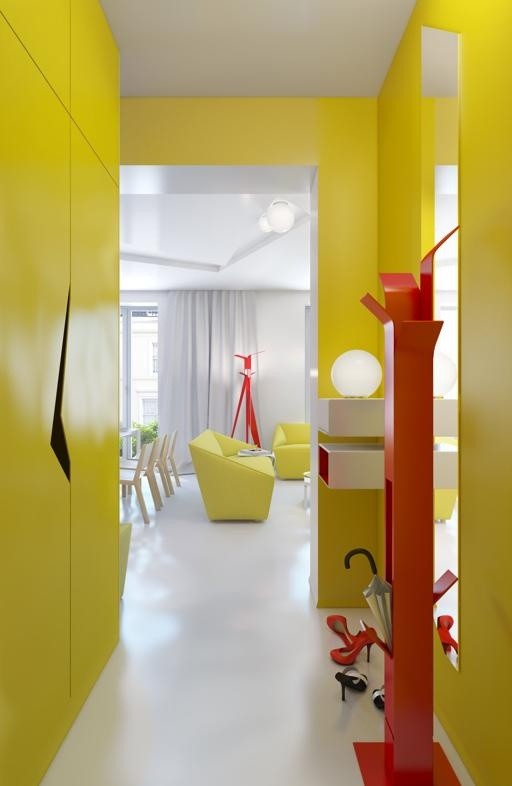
329, 347, 384, 405
257, 212, 275, 234
263, 199, 296, 234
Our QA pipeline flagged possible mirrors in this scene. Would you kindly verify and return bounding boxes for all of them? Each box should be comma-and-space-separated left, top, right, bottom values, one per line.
410, 20, 465, 673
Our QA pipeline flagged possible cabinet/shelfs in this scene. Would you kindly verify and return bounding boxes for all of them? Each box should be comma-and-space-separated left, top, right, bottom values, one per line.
314, 396, 386, 492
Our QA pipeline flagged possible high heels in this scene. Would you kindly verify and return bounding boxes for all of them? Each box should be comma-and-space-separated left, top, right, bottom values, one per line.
329, 627, 378, 667
371, 683, 385, 709
323, 613, 357, 648
436, 613, 458, 655
332, 665, 370, 703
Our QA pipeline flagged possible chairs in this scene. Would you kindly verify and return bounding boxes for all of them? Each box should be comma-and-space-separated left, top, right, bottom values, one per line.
116, 426, 183, 528
269, 416, 314, 482
186, 426, 277, 525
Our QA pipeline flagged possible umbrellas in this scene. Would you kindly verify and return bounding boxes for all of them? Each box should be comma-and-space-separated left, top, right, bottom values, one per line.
340, 544, 392, 661
